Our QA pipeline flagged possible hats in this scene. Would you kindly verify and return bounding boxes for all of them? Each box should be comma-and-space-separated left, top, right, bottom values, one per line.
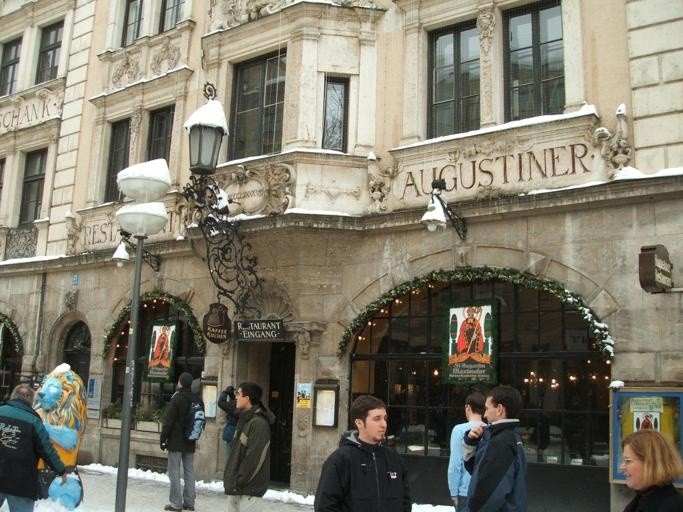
180, 373, 192, 388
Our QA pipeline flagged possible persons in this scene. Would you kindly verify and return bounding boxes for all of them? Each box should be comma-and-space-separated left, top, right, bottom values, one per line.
218, 386, 242, 464
447, 392, 488, 511
620, 431, 683, 512
0, 383, 67, 512
223, 381, 270, 512
641, 415, 653, 430
159, 372, 205, 512
457, 307, 484, 353
153, 327, 170, 360
462, 385, 527, 512
313, 395, 412, 512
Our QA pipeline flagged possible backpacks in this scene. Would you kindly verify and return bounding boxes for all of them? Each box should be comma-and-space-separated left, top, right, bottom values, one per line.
182, 391, 205, 442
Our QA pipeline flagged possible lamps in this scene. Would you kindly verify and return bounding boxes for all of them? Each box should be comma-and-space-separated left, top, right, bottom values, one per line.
111, 230, 161, 272
420, 179, 468, 240
183, 101, 240, 205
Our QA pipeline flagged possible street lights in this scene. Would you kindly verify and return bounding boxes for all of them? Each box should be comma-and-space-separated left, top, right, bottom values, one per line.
113, 152, 173, 512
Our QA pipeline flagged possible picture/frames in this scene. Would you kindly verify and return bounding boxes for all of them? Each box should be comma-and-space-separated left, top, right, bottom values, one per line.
143, 320, 181, 384
312, 379, 340, 428
441, 298, 499, 386
609, 386, 683, 488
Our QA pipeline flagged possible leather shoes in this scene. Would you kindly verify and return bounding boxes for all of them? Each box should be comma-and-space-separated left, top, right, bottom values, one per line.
165, 504, 182, 512
183, 504, 194, 510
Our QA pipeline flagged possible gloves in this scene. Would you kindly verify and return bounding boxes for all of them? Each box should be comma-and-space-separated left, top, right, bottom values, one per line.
159, 442, 167, 449
